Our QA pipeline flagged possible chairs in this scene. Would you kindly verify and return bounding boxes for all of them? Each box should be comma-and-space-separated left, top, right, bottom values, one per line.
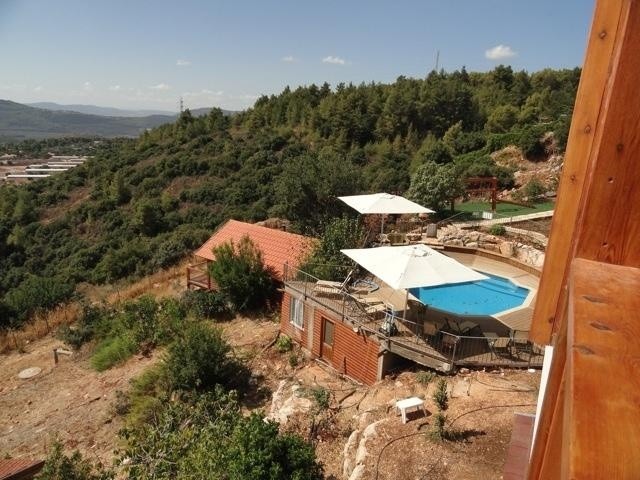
386, 233, 410, 246
406, 300, 532, 361
312, 269, 390, 323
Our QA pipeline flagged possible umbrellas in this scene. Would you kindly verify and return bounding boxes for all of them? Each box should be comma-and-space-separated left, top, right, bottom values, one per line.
340, 243, 490, 324
336, 192, 436, 234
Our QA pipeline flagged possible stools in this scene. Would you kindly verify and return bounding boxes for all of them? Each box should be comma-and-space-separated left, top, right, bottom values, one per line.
395, 397, 427, 424
